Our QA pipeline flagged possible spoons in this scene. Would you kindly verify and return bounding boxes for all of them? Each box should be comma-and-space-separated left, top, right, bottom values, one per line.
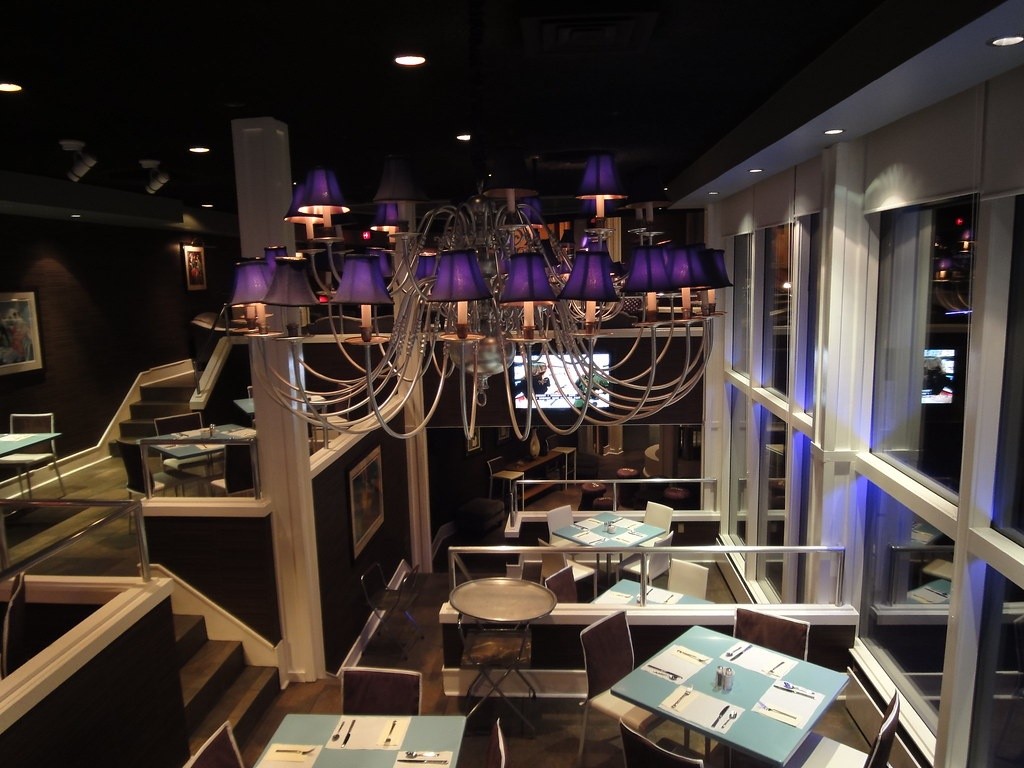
784, 681, 814, 695
726, 647, 742, 657
653, 671, 677, 680
406, 751, 439, 758
332, 721, 345, 741
721, 711, 737, 729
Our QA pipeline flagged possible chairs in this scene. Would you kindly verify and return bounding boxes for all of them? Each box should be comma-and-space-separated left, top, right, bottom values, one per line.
182, 501, 901, 768
994, 617, 1024, 757
10, 385, 314, 538
488, 432, 578, 509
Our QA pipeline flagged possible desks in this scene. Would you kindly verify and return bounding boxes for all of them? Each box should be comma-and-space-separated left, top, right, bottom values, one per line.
249, 714, 467, 768
618, 483, 649, 508
608, 625, 851, 767
552, 516, 667, 602
910, 518, 943, 544
905, 579, 952, 606
447, 576, 558, 732
138, 395, 327, 497
0, 432, 62, 459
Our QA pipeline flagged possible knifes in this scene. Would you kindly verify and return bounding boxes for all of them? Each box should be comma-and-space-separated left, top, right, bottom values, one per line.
648, 664, 683, 679
711, 705, 730, 728
398, 760, 448, 765
341, 720, 356, 748
730, 645, 753, 661
774, 685, 814, 699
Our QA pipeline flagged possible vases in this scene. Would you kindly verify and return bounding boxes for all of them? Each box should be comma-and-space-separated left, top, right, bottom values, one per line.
529, 427, 541, 461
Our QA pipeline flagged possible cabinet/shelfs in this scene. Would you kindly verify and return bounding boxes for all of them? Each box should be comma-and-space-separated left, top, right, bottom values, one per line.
506, 451, 564, 507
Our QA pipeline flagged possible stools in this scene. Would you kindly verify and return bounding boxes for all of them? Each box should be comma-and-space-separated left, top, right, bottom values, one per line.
573, 454, 600, 479
456, 498, 505, 537
578, 467, 692, 510
923, 558, 953, 579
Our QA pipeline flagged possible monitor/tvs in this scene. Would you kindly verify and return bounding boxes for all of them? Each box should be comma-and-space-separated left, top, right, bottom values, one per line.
511, 352, 613, 411
920, 347, 958, 406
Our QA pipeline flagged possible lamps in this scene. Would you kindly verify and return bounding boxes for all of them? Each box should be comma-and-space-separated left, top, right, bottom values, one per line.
936, 228, 976, 315
58, 0, 733, 442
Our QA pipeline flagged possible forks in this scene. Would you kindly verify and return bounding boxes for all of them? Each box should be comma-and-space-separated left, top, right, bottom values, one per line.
383, 720, 397, 746
677, 650, 709, 662
275, 747, 315, 755
759, 701, 797, 719
768, 662, 785, 675
671, 684, 693, 709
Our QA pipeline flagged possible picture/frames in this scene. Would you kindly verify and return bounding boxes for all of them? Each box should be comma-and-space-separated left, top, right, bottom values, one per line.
183, 244, 206, 291
348, 443, 384, 560
0, 292, 44, 376
